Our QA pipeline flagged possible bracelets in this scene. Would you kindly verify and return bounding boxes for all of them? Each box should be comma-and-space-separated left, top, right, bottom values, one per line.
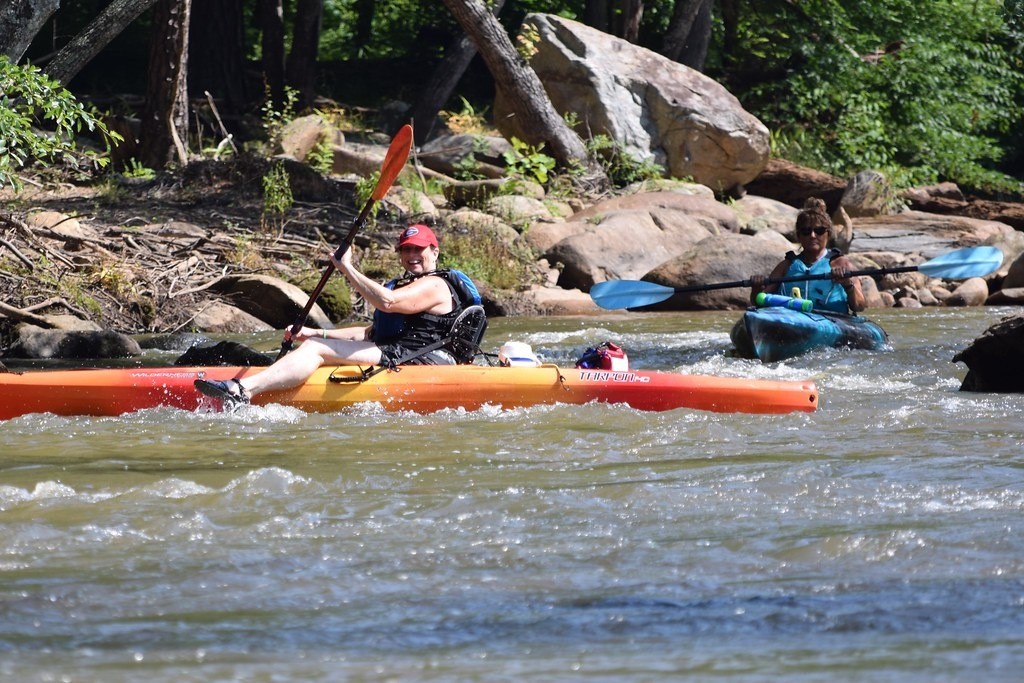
844, 281, 854, 291
323, 328, 327, 339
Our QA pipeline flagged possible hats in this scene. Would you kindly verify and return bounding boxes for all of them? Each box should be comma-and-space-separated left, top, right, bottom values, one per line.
395, 225, 437, 252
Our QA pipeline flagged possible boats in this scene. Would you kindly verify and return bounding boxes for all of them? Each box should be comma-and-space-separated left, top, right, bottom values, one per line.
0, 365, 818, 420
728, 306, 892, 362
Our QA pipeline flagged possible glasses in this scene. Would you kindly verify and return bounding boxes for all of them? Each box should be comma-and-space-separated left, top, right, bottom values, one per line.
401, 246, 422, 253
801, 226, 827, 235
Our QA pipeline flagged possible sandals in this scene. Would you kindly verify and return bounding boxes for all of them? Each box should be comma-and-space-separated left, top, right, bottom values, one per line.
194, 377, 250, 405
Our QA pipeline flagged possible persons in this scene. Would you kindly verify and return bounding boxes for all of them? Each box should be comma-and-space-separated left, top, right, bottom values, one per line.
194, 225, 465, 411
750, 197, 865, 317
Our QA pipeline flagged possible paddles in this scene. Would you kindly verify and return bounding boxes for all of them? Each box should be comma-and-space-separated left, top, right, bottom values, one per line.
275, 124, 414, 362
588, 246, 1003, 311
